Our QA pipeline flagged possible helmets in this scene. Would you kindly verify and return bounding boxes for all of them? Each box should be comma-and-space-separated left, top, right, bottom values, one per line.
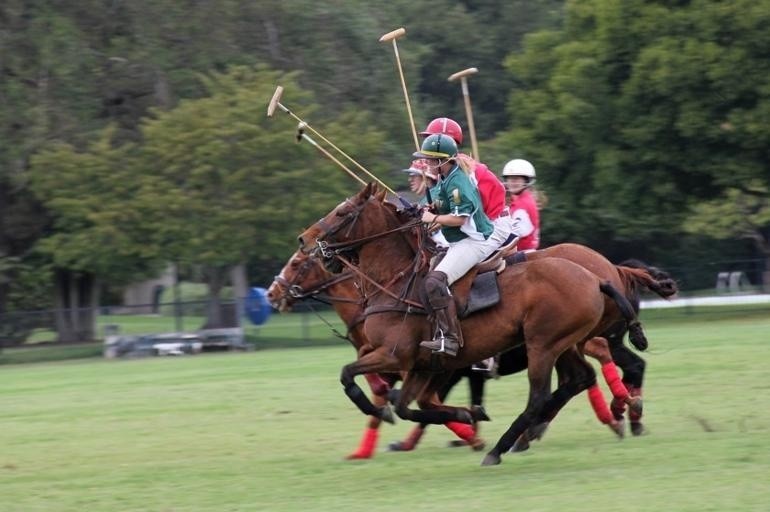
412, 133, 458, 160
417, 117, 462, 146
500, 159, 537, 178
402, 158, 439, 182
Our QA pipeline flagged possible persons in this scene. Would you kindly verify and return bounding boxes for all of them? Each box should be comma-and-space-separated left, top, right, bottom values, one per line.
402, 117, 512, 357
501, 158, 539, 251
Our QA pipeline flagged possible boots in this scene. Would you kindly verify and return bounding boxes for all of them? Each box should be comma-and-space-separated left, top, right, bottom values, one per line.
419, 295, 461, 357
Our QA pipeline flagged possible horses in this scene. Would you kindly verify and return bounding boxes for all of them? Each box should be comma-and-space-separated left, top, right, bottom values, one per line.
265, 179, 679, 468
